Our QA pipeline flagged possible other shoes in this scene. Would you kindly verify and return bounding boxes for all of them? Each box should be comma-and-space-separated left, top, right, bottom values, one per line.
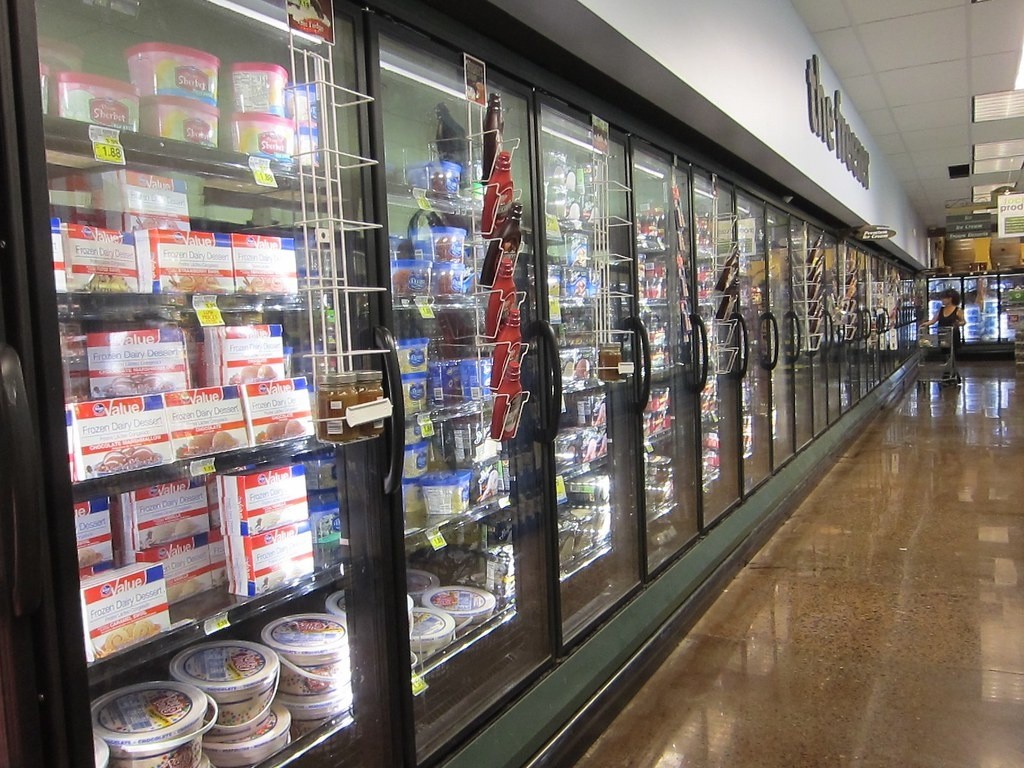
941, 372, 961, 386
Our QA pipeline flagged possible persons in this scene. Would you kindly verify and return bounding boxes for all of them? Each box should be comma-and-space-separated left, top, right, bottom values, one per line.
919, 289, 966, 387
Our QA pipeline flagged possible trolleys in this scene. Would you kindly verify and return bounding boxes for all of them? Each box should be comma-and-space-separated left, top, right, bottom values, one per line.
916, 323, 961, 392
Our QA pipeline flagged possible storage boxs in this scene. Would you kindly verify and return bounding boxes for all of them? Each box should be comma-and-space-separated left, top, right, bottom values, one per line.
44, 159, 472, 661
1006, 290, 1024, 379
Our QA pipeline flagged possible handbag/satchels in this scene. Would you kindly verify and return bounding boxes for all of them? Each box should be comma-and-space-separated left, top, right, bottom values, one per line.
955, 340, 973, 362
